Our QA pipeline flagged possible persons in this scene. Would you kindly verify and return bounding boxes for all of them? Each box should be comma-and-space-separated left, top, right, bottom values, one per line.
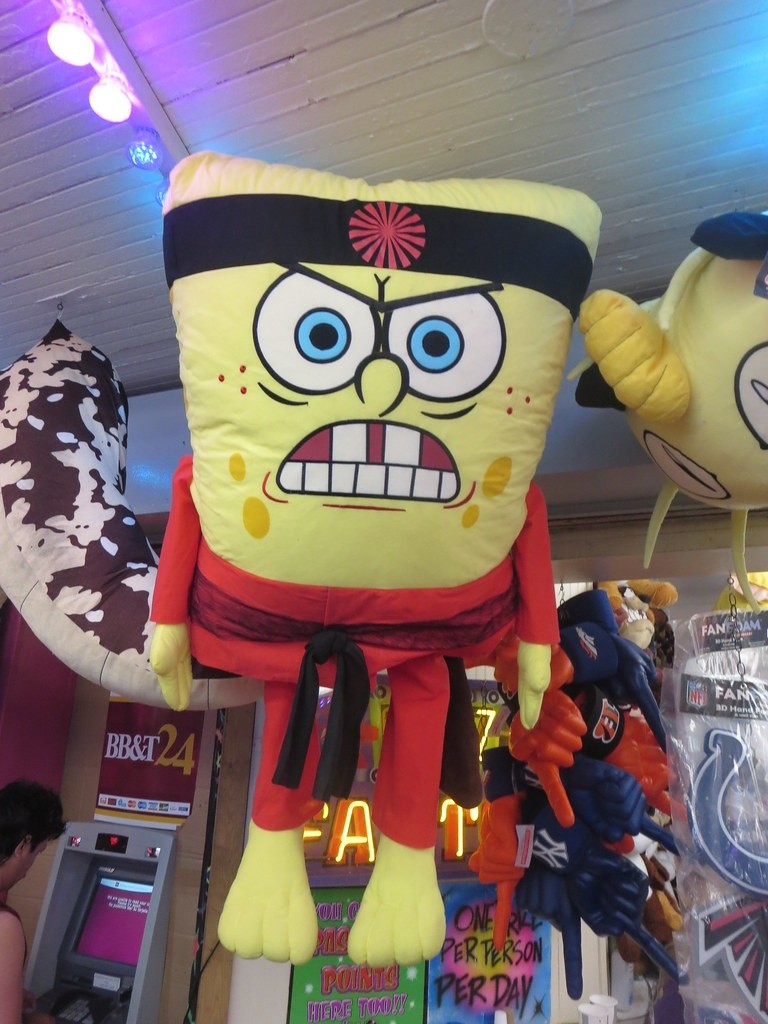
0, 778, 68, 1024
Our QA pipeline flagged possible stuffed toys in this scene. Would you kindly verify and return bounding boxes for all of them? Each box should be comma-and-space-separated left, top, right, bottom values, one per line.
1, 149, 768, 1001
